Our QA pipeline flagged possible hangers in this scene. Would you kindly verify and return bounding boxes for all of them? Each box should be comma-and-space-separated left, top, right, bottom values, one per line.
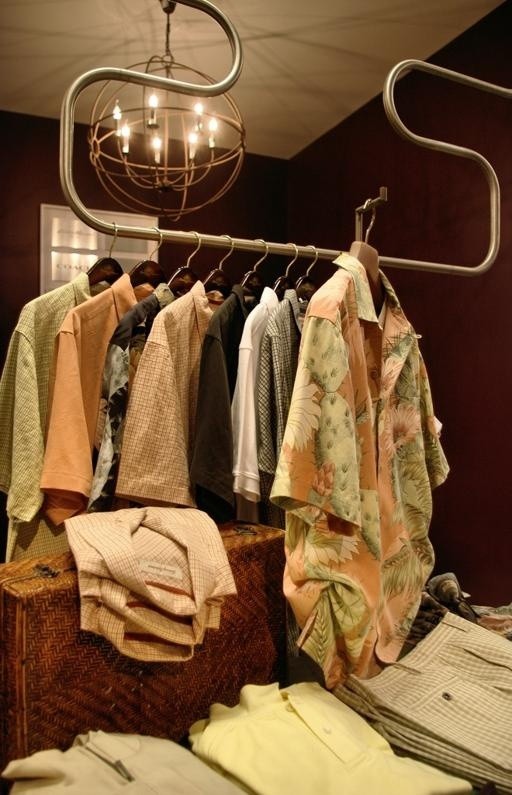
87, 199, 384, 312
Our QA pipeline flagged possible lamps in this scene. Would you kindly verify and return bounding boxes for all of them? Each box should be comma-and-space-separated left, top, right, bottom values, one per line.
88, 0, 247, 223
